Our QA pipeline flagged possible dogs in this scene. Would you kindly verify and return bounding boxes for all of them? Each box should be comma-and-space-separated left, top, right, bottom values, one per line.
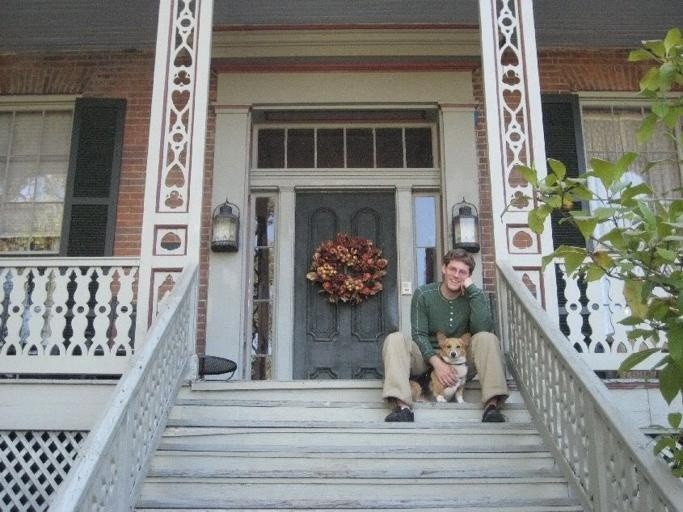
408, 330, 473, 404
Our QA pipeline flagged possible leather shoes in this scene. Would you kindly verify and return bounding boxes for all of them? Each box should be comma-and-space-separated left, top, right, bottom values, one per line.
384, 406, 414, 422
482, 408, 505, 422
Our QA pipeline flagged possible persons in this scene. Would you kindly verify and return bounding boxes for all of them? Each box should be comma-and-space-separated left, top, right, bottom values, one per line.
382, 248, 510, 422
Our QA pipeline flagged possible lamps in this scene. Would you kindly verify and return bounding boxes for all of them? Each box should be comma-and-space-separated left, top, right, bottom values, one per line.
450, 196, 480, 254
210, 198, 240, 252
